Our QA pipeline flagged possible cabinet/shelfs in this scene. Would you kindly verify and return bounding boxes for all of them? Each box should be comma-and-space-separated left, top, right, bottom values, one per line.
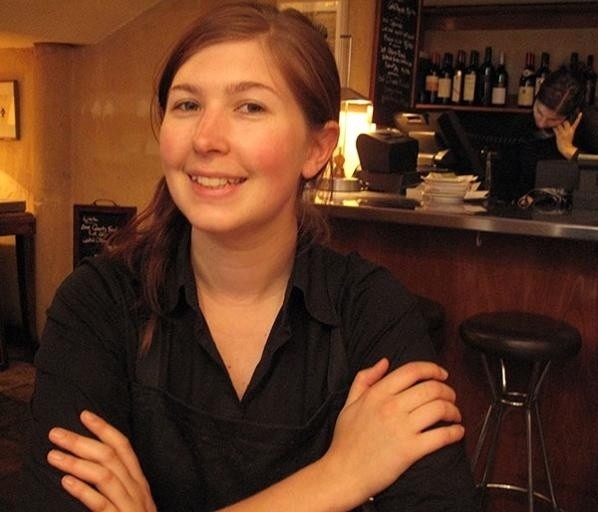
414, 0, 597, 115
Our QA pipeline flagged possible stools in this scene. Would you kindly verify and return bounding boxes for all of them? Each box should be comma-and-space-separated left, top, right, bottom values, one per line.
451, 307, 582, 511
408, 292, 443, 355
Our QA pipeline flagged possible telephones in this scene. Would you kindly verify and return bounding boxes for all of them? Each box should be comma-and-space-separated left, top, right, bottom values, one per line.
536, 106, 580, 140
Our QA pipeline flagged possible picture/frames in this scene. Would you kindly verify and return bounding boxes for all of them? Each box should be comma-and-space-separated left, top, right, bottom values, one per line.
0, 77, 21, 142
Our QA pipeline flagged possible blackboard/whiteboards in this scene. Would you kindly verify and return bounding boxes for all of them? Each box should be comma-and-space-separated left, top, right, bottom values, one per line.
371, 0, 421, 125
73, 198, 137, 272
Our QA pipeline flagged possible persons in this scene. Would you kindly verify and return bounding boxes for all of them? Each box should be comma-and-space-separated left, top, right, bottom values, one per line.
472, 67, 596, 203
17, 4, 477, 508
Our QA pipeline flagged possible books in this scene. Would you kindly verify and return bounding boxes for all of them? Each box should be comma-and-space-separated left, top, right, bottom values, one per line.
414, 171, 488, 216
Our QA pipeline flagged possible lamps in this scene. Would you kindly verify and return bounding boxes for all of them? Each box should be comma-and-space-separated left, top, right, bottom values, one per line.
332, 84, 374, 108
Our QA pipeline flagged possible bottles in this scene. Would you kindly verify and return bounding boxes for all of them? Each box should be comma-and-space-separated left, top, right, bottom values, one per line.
422, 48, 594, 108
330, 145, 345, 178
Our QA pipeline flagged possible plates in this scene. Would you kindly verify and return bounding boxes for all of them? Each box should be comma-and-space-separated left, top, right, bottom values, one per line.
418, 174, 471, 205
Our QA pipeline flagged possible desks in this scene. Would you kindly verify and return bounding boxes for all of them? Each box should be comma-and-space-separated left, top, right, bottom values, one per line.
0, 201, 38, 367
